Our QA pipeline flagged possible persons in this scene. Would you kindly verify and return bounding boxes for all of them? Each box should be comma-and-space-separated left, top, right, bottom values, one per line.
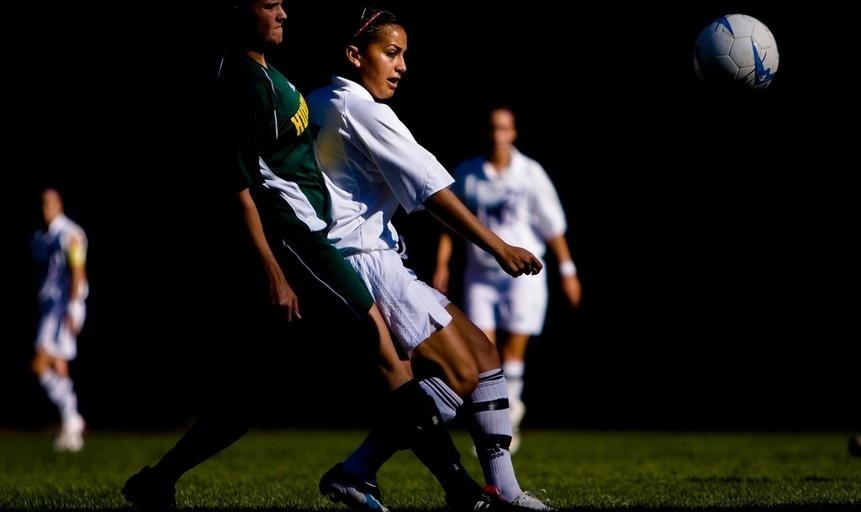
120, 0, 510, 509
431, 104, 585, 509
25, 189, 98, 460
308, 2, 554, 509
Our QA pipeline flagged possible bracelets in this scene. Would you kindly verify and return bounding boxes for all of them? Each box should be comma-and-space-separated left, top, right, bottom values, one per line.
558, 260, 578, 278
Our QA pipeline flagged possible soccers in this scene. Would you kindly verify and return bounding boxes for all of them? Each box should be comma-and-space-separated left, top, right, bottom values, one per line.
698, 15, 779, 93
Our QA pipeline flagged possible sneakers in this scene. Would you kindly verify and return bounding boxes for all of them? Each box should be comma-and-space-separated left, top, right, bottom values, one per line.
443, 489, 534, 512
490, 482, 557, 512
510, 399, 526, 456
121, 464, 180, 510
64, 414, 84, 453
319, 461, 390, 512
